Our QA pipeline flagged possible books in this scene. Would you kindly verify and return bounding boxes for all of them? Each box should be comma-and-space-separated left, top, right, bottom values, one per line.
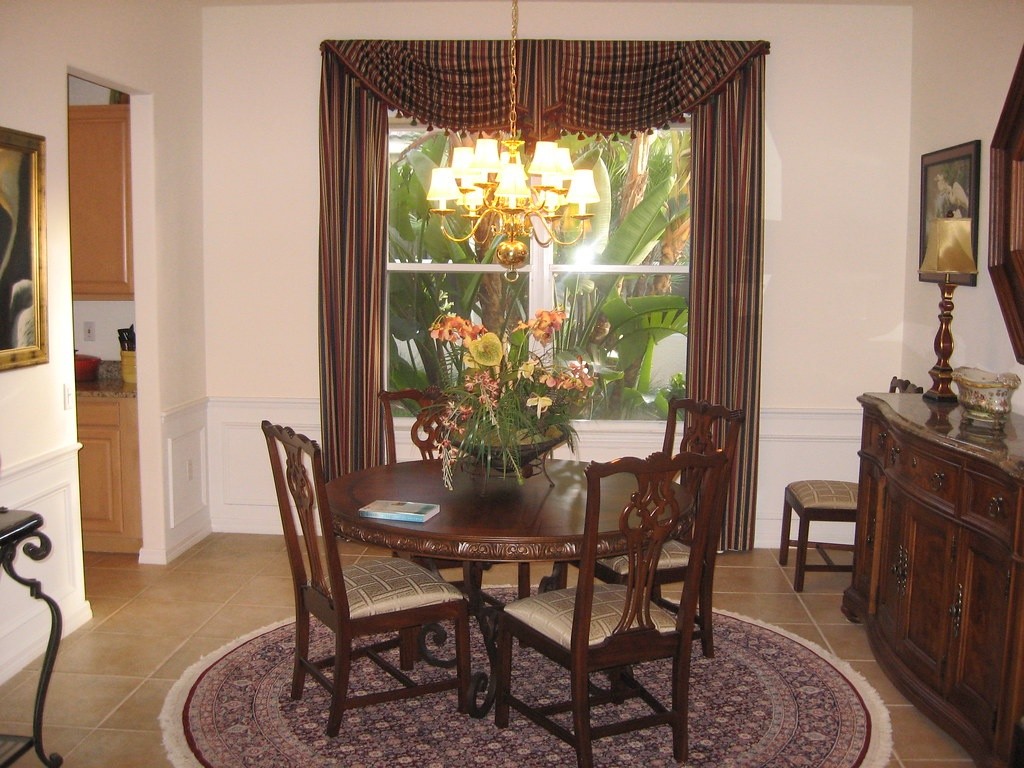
358, 500, 440, 522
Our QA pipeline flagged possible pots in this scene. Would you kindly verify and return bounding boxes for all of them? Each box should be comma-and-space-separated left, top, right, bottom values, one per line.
75, 349, 101, 381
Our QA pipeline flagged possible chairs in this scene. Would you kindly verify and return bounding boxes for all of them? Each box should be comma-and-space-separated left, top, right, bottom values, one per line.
541, 392, 749, 660
260, 419, 474, 734
779, 375, 921, 590
494, 447, 729, 768
372, 379, 533, 650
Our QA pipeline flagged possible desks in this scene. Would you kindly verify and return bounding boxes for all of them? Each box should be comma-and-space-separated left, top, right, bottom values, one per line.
0, 507, 67, 768
327, 457, 695, 717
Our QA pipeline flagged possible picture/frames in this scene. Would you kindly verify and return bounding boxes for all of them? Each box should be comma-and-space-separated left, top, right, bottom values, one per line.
918, 141, 981, 286
0, 119, 49, 374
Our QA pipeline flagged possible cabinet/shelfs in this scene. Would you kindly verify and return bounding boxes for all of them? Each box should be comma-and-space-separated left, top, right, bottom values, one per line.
839, 391, 1024, 768
68, 104, 133, 301
74, 399, 138, 555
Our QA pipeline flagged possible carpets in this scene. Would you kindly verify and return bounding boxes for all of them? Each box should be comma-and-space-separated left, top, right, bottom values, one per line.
159, 581, 892, 768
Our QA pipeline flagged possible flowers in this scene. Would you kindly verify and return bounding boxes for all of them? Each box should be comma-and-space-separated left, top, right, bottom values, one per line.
427, 310, 598, 492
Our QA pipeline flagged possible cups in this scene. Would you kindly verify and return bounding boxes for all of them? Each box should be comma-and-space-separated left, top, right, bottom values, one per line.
121, 351, 137, 383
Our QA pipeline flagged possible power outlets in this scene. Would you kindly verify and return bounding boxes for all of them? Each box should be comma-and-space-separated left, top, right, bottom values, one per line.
187, 459, 194, 483
84, 321, 95, 341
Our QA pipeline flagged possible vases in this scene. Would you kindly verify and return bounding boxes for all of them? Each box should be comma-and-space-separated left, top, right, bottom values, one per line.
454, 429, 570, 493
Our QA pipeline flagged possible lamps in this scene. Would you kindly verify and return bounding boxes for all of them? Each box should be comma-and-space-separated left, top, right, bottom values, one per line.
424, 0, 600, 283
